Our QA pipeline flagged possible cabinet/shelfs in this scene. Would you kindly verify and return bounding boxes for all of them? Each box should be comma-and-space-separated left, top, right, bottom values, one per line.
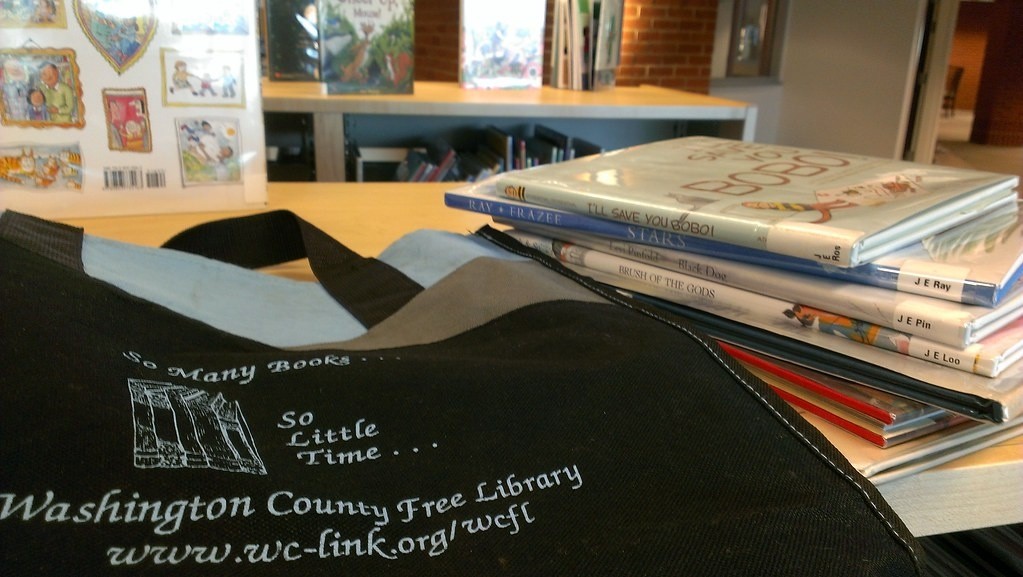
49, 183, 1023, 539
263, 77, 759, 182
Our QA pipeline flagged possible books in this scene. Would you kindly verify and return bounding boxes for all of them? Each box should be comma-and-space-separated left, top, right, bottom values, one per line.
397, 132, 1022, 449
457, 0, 547, 94
549, 0, 624, 91
261, 0, 416, 99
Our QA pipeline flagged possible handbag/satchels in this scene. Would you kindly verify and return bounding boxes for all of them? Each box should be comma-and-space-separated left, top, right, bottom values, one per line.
0, 209, 931, 577
